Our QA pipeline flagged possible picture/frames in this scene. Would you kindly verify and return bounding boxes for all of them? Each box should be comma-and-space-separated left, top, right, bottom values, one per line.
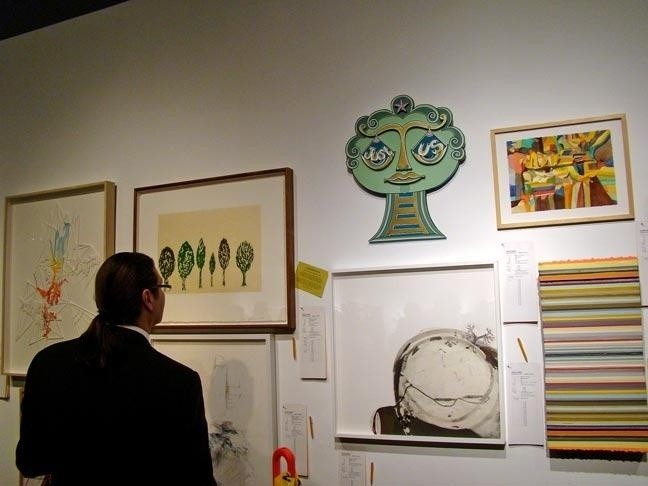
1, 181, 115, 379
133, 167, 295, 334
149, 335, 278, 485
490, 113, 635, 231
332, 262, 507, 447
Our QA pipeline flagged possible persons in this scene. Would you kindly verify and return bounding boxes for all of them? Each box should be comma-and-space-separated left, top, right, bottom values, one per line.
12, 251, 219, 484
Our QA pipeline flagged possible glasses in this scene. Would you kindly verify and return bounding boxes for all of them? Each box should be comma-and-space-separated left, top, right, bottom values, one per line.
156, 283, 172, 293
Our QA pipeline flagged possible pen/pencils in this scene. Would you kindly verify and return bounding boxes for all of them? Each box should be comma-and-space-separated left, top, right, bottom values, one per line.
310, 416, 313, 438
517, 338, 528, 362
293, 337, 295, 359
371, 462, 373, 485
